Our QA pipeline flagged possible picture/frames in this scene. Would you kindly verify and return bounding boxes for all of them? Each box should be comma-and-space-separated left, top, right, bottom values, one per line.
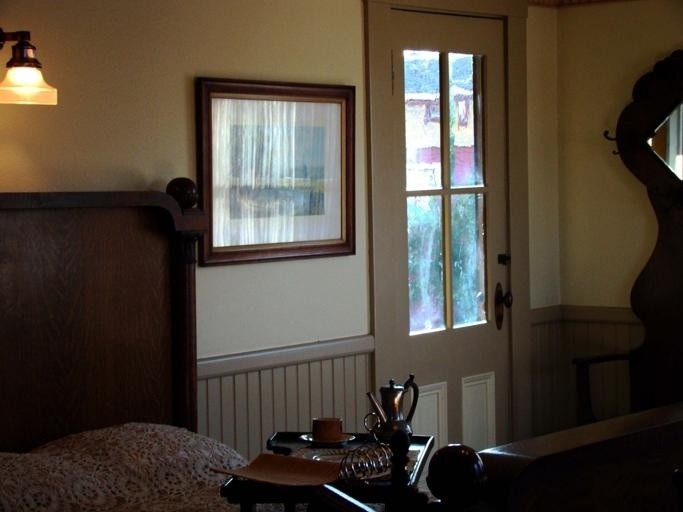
191, 72, 360, 266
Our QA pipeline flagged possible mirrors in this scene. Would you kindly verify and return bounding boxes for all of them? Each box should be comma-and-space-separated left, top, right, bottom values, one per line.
616, 49, 683, 357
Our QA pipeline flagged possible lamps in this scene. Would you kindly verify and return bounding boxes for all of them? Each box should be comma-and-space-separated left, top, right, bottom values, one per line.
0, 28, 58, 105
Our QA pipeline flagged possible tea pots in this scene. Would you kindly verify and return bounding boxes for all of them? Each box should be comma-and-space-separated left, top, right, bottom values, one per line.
363, 372, 420, 458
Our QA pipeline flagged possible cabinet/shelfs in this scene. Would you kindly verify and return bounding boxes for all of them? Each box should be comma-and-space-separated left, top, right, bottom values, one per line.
478, 404, 682, 512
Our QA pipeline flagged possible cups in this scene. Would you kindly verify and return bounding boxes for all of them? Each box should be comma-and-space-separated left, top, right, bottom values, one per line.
312, 417, 343, 442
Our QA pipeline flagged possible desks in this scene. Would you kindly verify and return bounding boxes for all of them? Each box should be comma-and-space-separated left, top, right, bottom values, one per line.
221, 428, 435, 512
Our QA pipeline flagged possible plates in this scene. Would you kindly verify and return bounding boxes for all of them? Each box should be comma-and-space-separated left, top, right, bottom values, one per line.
299, 432, 356, 442
296, 432, 356, 444
221, 430, 435, 503
285, 447, 392, 481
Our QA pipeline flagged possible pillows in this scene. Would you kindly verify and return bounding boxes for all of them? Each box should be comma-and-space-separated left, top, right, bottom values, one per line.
2, 421, 250, 512
